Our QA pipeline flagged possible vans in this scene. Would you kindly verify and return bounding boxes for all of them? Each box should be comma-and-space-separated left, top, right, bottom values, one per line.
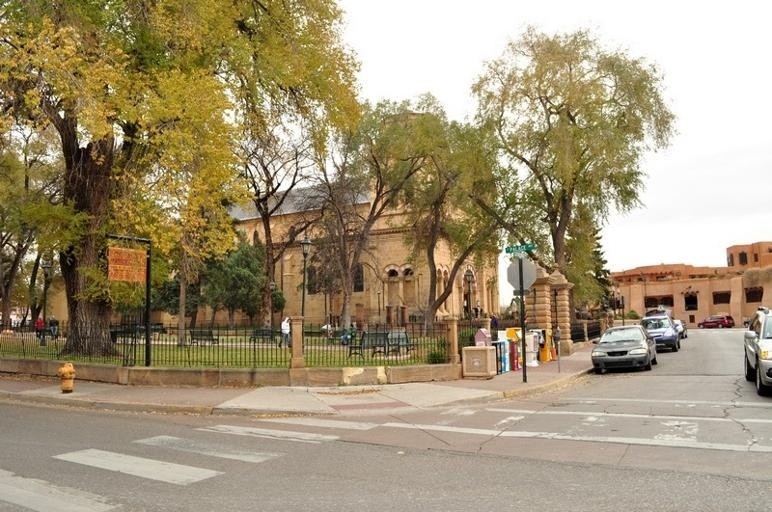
698, 315, 735, 329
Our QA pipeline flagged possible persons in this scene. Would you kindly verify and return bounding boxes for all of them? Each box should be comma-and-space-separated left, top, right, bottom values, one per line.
35, 318, 44, 339
49, 316, 59, 340
278, 317, 292, 348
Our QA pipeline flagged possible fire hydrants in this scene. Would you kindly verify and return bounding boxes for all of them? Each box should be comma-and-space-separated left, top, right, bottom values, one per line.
57, 363, 75, 394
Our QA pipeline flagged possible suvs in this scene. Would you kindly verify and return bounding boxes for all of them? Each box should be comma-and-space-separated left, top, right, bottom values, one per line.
743, 306, 772, 396
641, 316, 681, 351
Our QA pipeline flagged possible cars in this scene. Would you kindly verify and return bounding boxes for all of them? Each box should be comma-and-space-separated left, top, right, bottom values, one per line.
591, 326, 658, 373
674, 320, 688, 337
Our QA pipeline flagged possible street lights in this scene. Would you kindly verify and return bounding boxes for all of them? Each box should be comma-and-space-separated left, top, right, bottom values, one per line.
299, 227, 311, 356
464, 270, 475, 317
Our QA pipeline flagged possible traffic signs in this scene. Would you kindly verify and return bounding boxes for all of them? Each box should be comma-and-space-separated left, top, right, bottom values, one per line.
506, 243, 536, 253
